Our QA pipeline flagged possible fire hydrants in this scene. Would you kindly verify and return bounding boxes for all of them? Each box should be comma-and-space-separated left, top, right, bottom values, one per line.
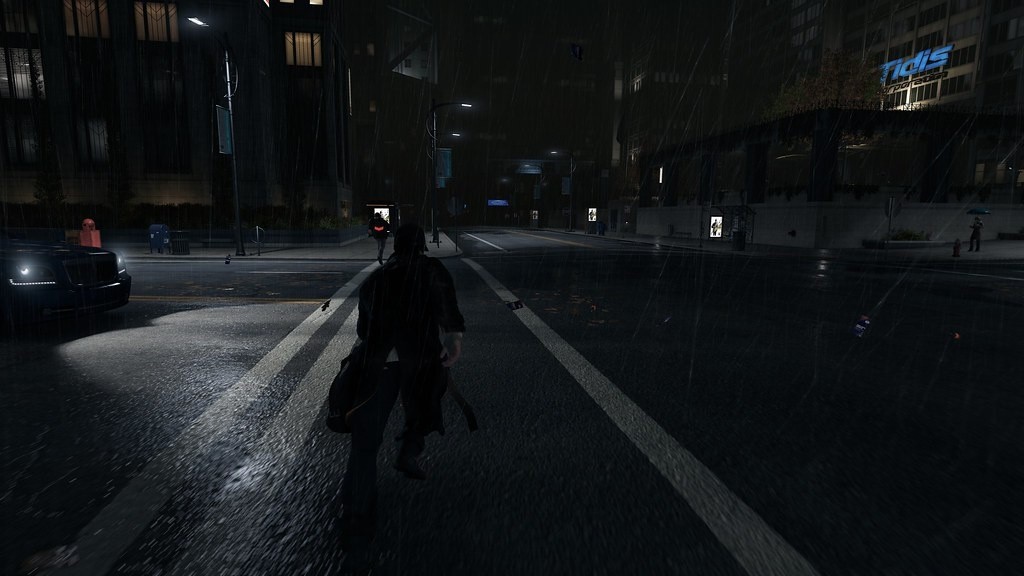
952, 238, 961, 257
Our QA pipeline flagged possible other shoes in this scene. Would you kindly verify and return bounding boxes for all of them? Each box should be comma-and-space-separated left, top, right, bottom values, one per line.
378, 257, 383, 265
339, 469, 377, 503
390, 448, 427, 487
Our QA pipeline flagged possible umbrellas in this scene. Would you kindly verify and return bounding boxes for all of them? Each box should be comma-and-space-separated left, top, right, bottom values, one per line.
966, 207, 991, 225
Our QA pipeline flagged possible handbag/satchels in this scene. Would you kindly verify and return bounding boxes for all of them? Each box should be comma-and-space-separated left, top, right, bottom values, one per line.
326, 339, 396, 434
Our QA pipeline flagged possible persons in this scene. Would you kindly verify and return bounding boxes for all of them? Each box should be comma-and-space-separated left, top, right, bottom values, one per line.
335, 222, 467, 543
371, 213, 389, 265
968, 217, 983, 252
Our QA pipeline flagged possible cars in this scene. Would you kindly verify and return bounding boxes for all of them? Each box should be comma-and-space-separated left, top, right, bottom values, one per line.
0, 239, 132, 331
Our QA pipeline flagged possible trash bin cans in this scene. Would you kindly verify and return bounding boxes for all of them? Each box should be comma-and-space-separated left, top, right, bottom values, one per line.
149, 224, 171, 254
169, 230, 190, 255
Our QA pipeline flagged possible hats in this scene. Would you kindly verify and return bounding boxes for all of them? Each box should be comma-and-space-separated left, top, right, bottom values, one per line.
374, 213, 381, 217
395, 222, 429, 253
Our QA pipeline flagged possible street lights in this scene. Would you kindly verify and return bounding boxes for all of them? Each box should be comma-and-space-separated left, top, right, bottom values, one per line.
551, 151, 574, 232
186, 17, 250, 255
423, 103, 472, 243
